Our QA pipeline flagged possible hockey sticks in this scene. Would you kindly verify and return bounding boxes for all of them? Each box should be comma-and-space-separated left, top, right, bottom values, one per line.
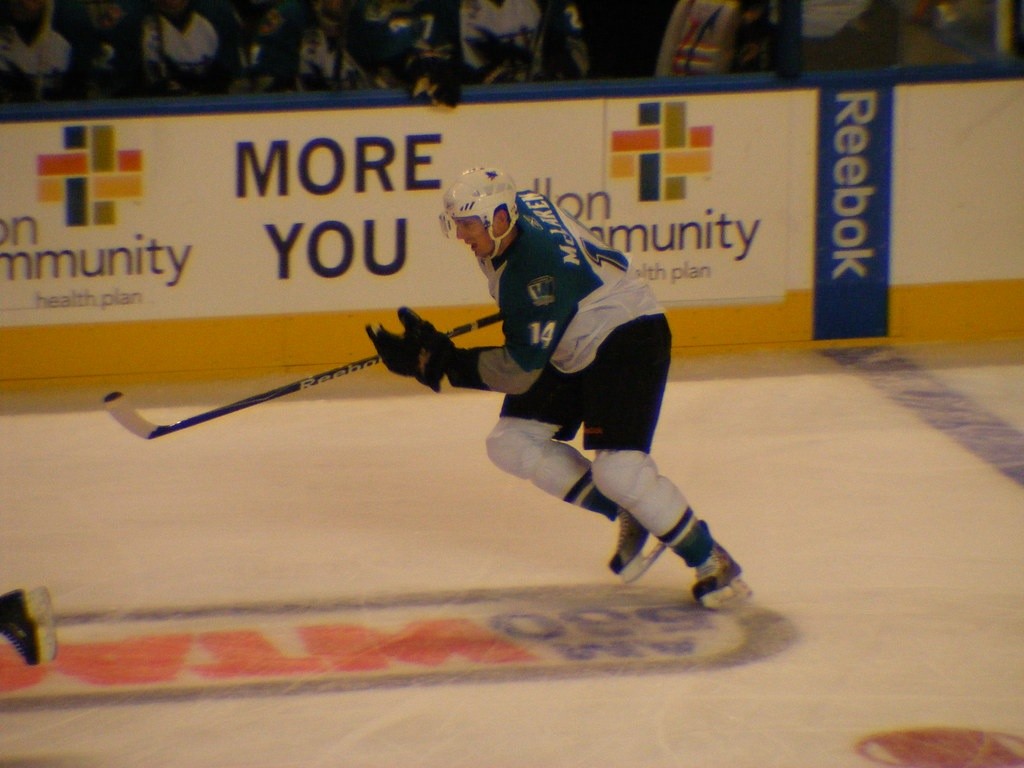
100, 311, 504, 442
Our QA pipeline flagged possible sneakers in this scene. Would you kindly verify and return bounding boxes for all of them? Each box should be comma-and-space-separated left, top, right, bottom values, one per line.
676, 520, 753, 609
0, 585, 57, 666
609, 506, 666, 584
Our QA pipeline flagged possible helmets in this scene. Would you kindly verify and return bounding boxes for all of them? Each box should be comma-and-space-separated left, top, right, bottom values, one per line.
443, 165, 518, 241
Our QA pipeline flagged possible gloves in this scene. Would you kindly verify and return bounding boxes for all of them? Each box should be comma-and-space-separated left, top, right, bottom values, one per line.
365, 308, 455, 394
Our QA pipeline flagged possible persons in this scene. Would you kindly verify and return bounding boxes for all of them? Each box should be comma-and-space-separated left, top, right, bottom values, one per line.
365, 166, 751, 610
0, 0, 590, 109
0, 586, 57, 668
